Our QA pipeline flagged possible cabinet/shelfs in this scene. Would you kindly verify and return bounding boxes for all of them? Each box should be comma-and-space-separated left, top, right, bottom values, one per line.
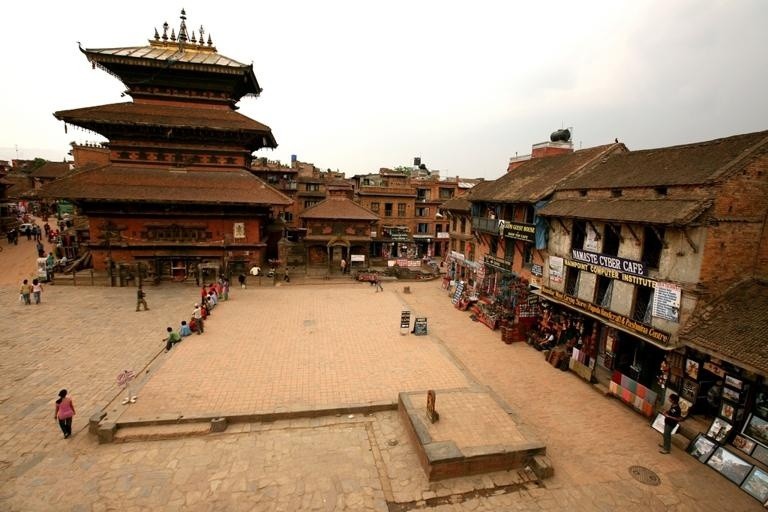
477, 302, 514, 330
36, 257, 48, 283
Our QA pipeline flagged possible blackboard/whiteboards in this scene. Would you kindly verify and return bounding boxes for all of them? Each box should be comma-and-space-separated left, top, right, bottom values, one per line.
401, 311, 410, 328
451, 282, 465, 305
415, 318, 427, 335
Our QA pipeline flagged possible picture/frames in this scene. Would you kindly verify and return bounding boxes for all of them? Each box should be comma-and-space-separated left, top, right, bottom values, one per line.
650, 359, 767, 504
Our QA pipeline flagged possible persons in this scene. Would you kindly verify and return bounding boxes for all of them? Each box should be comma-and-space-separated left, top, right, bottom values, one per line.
239, 272, 248, 290
162, 271, 230, 352
340, 257, 345, 270
375, 270, 383, 293
539, 330, 554, 349
20, 278, 32, 305
53, 388, 76, 439
284, 267, 289, 283
135, 285, 150, 312
267, 267, 276, 277
658, 394, 681, 454
0, 206, 73, 281
249, 264, 260, 276
30, 278, 43, 305
